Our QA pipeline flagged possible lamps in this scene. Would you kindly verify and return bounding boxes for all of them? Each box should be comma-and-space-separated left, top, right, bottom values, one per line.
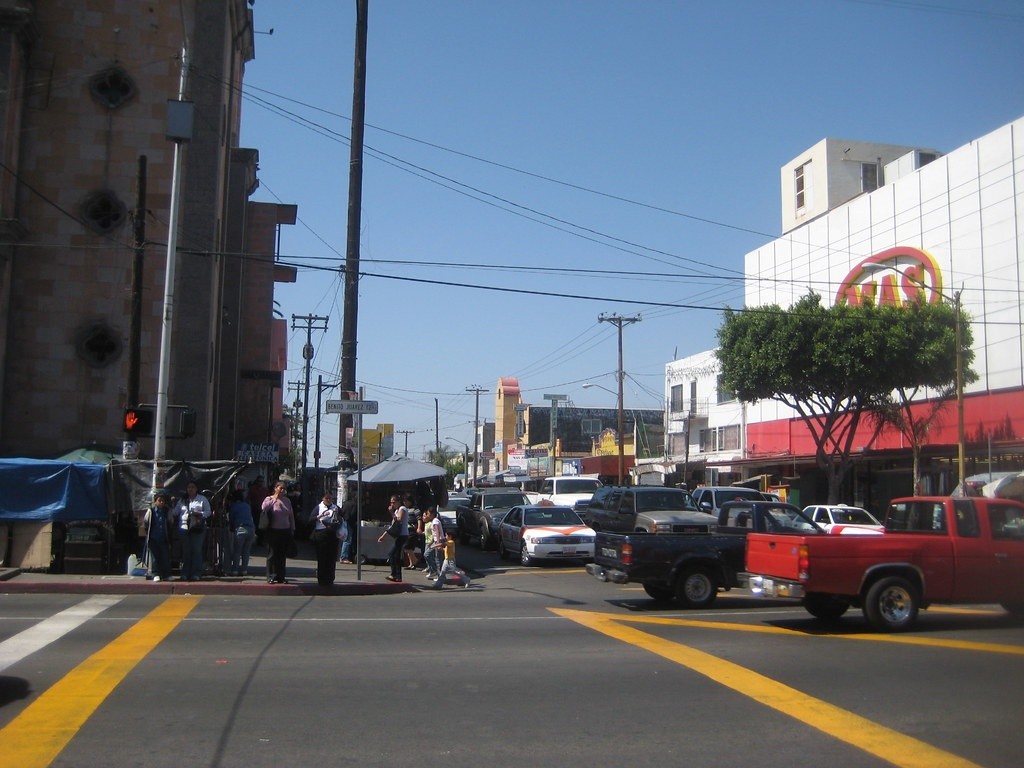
254, 28, 274, 35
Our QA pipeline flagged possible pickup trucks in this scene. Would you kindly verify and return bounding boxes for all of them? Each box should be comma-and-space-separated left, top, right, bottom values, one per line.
585, 502, 830, 605
456, 490, 533, 549
737, 494, 1024, 633
524, 476, 604, 512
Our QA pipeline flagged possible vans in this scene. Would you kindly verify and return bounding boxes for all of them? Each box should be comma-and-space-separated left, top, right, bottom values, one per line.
942, 471, 1019, 521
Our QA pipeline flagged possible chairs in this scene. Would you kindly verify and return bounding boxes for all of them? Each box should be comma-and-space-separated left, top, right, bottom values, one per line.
551, 512, 564, 523
735, 512, 751, 527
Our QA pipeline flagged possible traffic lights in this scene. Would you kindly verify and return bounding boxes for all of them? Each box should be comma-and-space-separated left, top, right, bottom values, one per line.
123, 409, 152, 437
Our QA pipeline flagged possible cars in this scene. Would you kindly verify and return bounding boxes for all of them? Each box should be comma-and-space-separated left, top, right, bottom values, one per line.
781, 502, 886, 535
447, 487, 481, 499
499, 505, 598, 564
436, 498, 471, 533
761, 492, 785, 515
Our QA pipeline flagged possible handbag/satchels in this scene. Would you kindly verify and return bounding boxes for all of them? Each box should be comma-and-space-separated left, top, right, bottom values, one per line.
186, 497, 205, 532
286, 535, 298, 557
321, 507, 343, 530
255, 495, 274, 535
387, 520, 402, 538
336, 518, 348, 542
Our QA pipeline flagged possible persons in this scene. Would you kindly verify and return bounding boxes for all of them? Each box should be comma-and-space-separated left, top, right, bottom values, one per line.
377, 494, 410, 582
337, 495, 364, 564
144, 493, 176, 581
261, 482, 295, 584
403, 493, 447, 581
172, 480, 211, 580
309, 489, 345, 585
430, 530, 471, 588
248, 475, 270, 545
229, 491, 255, 575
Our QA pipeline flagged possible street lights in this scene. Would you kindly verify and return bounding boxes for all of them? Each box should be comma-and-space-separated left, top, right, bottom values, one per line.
582, 384, 625, 487
149, 100, 199, 487
445, 436, 468, 489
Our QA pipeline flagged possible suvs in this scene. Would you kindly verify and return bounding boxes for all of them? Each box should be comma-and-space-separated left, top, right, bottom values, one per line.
583, 487, 720, 533
689, 486, 791, 528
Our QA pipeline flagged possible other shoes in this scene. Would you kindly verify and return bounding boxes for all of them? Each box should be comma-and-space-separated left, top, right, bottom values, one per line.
385, 575, 402, 581
181, 575, 188, 581
339, 559, 353, 565
267, 577, 288, 584
404, 563, 416, 571
153, 576, 160, 582
168, 576, 181, 581
192, 575, 200, 581
232, 570, 247, 576
422, 567, 471, 590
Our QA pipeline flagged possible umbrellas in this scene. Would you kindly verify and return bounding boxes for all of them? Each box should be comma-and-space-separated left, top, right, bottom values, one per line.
346, 452, 448, 501
